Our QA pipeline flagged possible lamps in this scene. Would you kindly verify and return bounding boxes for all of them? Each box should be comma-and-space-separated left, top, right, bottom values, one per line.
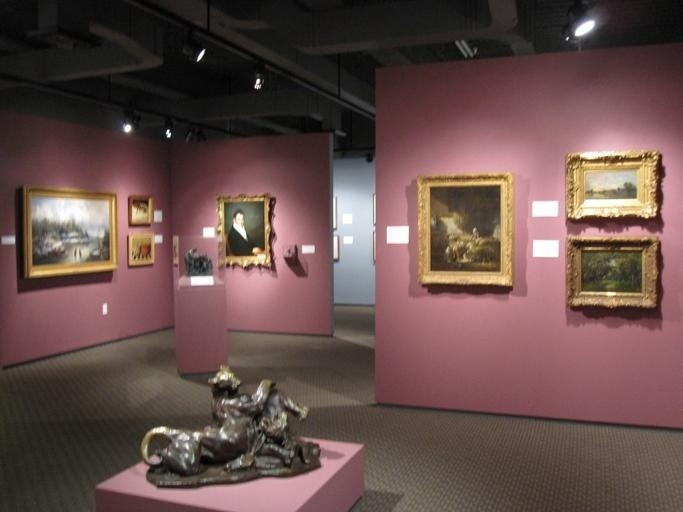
565, 1, 598, 40
161, 118, 173, 141
122, 111, 140, 134
248, 57, 267, 96
176, 24, 209, 63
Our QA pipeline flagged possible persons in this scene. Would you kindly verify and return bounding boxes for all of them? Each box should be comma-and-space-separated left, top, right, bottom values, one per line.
226, 208, 266, 257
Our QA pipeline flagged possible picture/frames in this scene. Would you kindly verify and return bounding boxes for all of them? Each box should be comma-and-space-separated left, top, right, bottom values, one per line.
214, 191, 273, 267
416, 173, 515, 288
127, 195, 155, 267
566, 233, 661, 311
21, 183, 117, 280
565, 151, 663, 222
